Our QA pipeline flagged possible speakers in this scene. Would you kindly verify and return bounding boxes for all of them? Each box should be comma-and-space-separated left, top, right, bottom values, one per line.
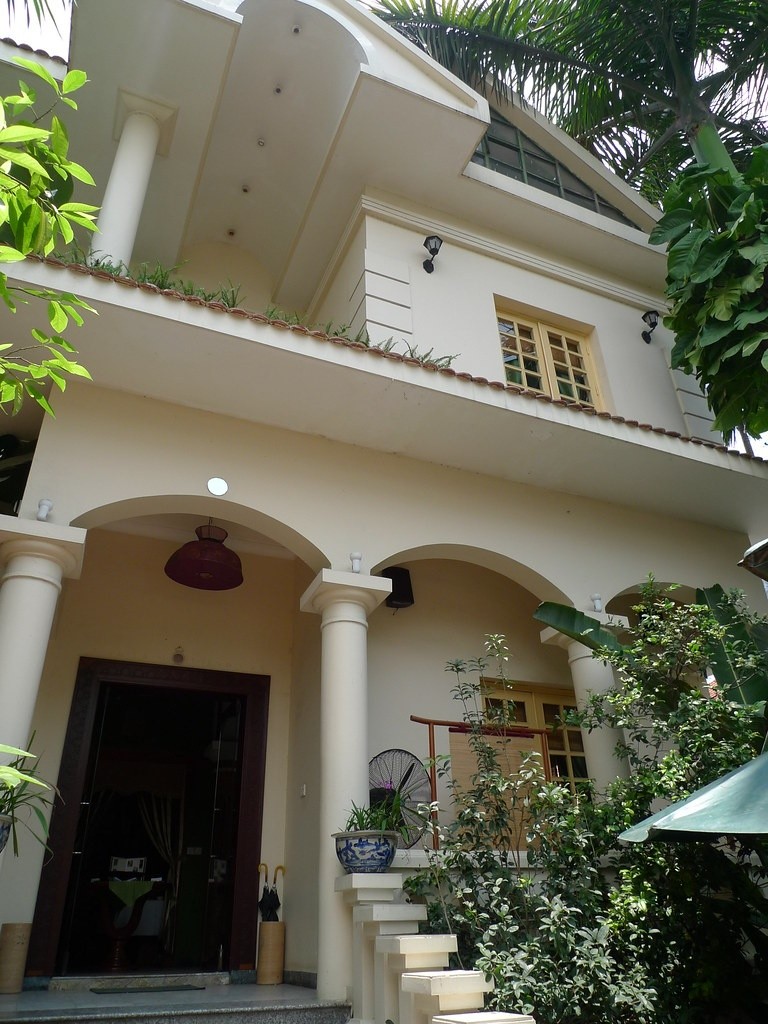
383, 566, 415, 608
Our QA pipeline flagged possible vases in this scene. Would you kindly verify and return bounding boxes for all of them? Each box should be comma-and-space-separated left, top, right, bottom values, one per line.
331, 831, 401, 875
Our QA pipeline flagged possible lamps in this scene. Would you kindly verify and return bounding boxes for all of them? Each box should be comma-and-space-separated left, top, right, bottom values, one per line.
422, 235, 444, 275
162, 516, 245, 591
225, 26, 300, 239
641, 310, 659, 345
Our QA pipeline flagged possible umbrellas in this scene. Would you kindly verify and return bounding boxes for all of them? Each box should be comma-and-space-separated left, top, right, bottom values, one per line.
258, 862, 286, 922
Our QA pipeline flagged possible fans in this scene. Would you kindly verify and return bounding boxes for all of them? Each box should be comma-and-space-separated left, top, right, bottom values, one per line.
368, 748, 432, 849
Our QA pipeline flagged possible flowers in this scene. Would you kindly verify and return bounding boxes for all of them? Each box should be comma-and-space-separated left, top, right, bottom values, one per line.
328, 780, 419, 844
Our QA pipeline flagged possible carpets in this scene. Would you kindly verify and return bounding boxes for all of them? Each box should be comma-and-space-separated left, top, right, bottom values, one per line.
89, 984, 207, 994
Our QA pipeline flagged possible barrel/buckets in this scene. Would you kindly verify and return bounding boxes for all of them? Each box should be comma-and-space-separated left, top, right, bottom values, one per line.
256, 922, 284, 984
